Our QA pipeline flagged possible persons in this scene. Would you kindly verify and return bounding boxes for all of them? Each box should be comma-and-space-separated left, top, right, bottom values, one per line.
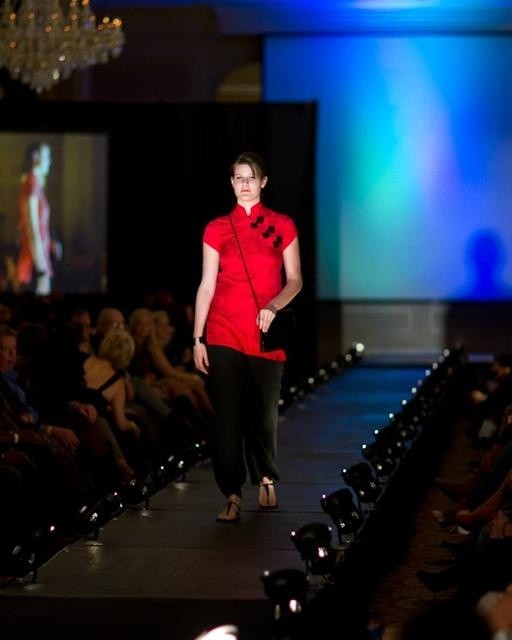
1, 287, 218, 574
431, 352, 512, 640
15, 140, 56, 295
193, 151, 304, 524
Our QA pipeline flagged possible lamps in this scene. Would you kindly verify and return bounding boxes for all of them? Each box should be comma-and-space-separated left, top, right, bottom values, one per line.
5, 438, 210, 584
261, 401, 424, 621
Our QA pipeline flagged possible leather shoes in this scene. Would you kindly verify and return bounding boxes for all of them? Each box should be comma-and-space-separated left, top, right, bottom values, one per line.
258, 482, 278, 510
216, 498, 241, 522
414, 570, 448, 594
433, 510, 481, 535
433, 476, 464, 504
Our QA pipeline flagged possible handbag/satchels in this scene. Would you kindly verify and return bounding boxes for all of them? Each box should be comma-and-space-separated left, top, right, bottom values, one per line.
259, 308, 298, 352
